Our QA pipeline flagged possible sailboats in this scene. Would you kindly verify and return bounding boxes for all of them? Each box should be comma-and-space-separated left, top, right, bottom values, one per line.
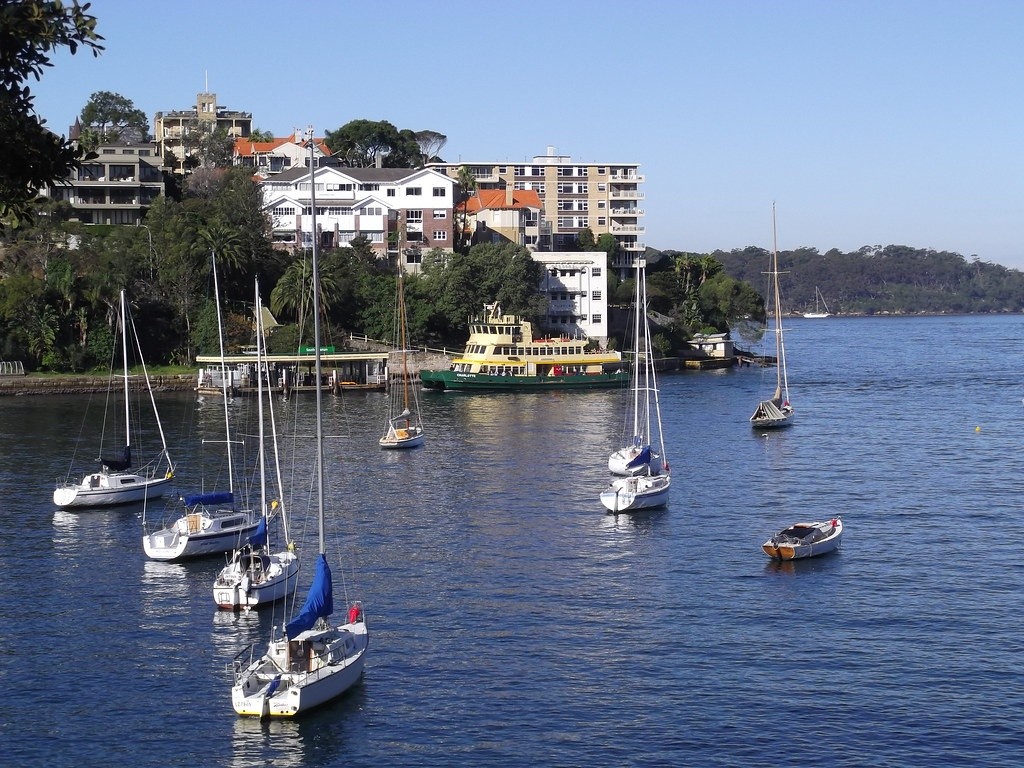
210, 271, 301, 607
140, 248, 269, 561
749, 200, 795, 426
227, 141, 371, 721
803, 286, 830, 318
599, 258, 672, 513
379, 230, 426, 451
52, 287, 179, 506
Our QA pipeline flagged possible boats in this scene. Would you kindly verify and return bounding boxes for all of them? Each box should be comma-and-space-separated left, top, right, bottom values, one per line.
761, 516, 845, 561
418, 300, 634, 391
193, 344, 391, 395
